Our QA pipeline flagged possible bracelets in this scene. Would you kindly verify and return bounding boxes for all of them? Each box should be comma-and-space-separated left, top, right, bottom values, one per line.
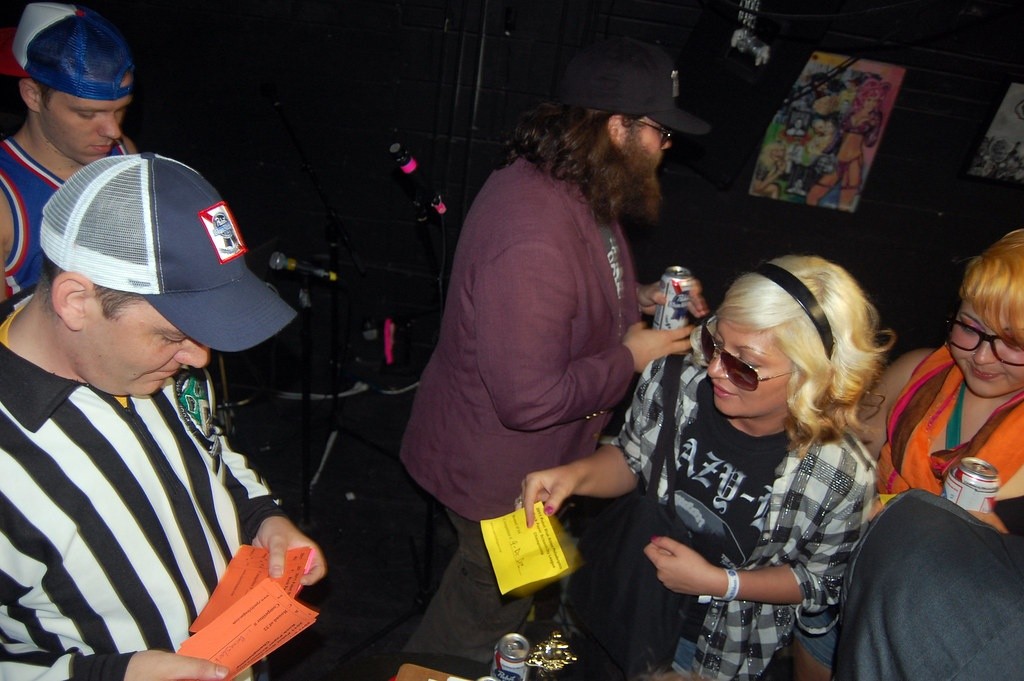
713, 568, 740, 602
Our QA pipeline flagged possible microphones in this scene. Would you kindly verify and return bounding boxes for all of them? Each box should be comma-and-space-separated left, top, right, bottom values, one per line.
389, 143, 445, 215
267, 252, 338, 282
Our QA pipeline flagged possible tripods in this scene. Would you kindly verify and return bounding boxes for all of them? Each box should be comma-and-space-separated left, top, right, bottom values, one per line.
270, 99, 450, 664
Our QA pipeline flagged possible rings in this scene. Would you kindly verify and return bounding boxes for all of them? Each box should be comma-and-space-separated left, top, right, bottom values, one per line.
514, 499, 523, 508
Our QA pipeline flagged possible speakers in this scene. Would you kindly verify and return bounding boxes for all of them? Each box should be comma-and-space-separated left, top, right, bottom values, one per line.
674, 0, 846, 192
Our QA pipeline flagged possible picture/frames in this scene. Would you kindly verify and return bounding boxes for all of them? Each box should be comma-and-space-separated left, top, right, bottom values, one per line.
956, 77, 1024, 189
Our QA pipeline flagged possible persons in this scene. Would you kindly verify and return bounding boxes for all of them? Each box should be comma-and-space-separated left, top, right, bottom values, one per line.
0, 3, 140, 304
399, 35, 711, 662
793, 229, 1024, 681
505, 254, 887, 681
0, 156, 328, 681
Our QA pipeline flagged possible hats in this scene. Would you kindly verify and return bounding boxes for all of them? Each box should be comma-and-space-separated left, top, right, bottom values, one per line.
0, 2, 137, 100
40, 152, 298, 352
560, 38, 712, 134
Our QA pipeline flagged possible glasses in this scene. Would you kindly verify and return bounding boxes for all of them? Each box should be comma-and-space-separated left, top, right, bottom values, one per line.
701, 310, 797, 390
944, 300, 1024, 366
628, 118, 672, 146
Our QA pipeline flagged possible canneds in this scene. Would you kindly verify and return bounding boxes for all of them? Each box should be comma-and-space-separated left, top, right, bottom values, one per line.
652, 265, 693, 329
942, 457, 1001, 513
490, 633, 531, 681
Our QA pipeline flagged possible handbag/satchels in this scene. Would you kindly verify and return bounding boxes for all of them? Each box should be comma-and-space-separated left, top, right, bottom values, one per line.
565, 490, 700, 681
